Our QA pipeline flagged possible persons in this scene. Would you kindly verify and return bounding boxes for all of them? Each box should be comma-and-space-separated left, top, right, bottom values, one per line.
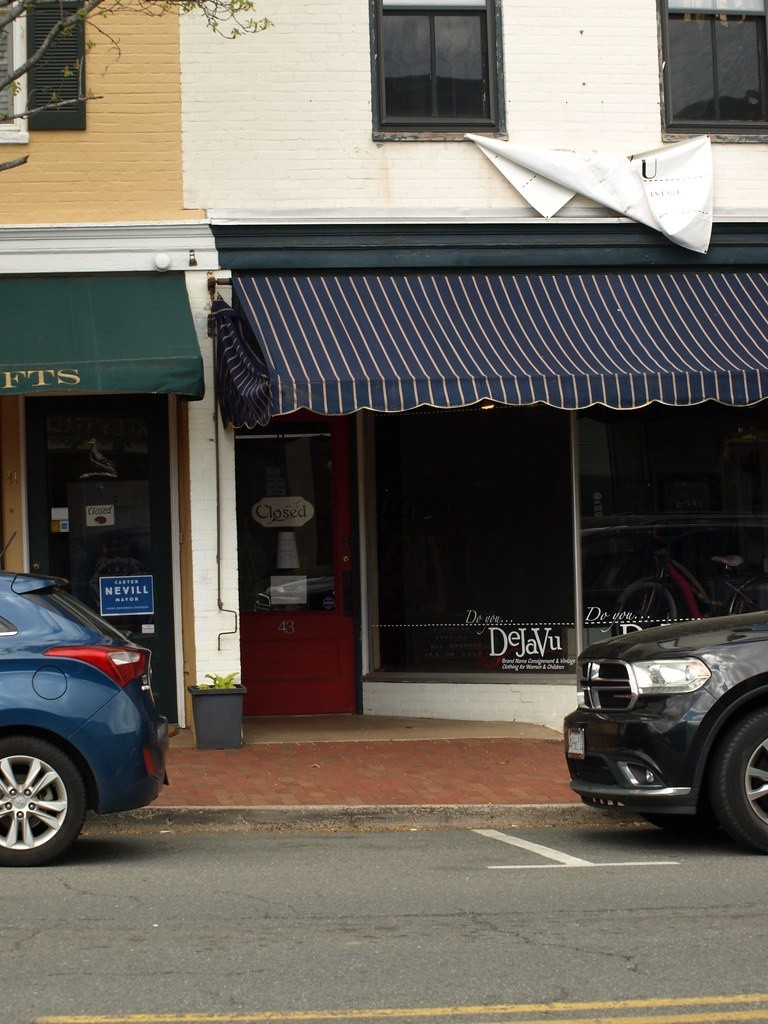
408, 470, 448, 615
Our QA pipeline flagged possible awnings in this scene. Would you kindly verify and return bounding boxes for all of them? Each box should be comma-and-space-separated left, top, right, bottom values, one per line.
0, 270, 206, 405
212, 272, 768, 428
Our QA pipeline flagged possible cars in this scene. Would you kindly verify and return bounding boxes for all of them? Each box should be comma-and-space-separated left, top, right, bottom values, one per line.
563, 606, 768, 853
0, 571, 168, 863
253, 563, 337, 612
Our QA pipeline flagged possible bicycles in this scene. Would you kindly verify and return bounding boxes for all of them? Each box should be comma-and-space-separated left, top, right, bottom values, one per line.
613, 525, 768, 638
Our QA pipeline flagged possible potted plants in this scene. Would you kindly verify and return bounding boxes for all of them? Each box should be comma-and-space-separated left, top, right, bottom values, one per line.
187, 671, 247, 750
